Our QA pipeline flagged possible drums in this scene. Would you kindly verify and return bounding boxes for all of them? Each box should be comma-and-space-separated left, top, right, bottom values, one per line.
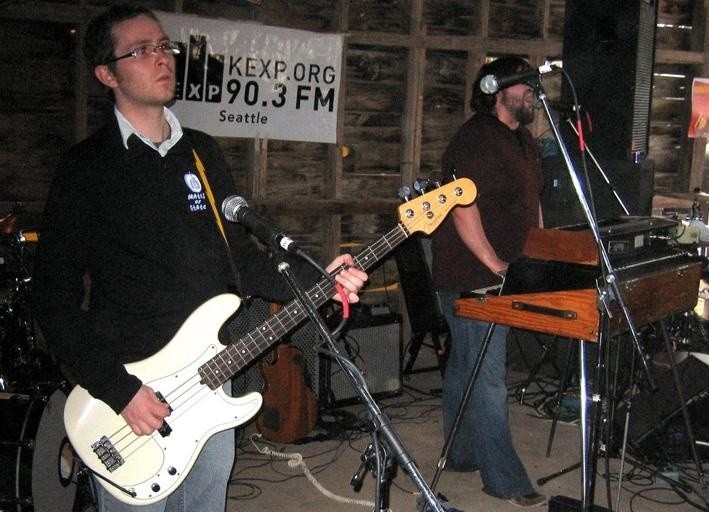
1, 375, 81, 512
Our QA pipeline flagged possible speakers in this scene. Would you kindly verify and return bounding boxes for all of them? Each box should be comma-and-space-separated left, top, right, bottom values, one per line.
325, 301, 403, 409
613, 355, 709, 462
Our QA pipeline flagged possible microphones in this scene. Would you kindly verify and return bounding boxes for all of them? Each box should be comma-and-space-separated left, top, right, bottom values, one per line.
532, 98, 582, 112
479, 64, 556, 94
221, 195, 301, 257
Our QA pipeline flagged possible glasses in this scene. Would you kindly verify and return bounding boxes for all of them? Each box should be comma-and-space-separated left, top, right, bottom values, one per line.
102, 41, 180, 64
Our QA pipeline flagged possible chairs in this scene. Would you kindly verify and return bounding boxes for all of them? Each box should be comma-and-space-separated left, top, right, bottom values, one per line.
396, 239, 447, 377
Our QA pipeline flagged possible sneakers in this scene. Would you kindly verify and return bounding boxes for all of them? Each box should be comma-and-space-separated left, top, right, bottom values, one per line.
506, 492, 546, 508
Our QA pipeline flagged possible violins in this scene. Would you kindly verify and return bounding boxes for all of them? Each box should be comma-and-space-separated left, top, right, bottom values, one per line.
256, 302, 319, 444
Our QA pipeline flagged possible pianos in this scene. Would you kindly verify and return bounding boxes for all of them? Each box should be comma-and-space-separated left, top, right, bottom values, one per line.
453, 215, 703, 344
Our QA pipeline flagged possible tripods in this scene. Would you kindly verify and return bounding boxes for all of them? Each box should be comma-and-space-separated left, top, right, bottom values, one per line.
522, 333, 574, 458
537, 334, 691, 495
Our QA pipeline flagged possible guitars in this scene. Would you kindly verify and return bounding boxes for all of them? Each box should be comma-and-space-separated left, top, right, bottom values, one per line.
64, 167, 477, 507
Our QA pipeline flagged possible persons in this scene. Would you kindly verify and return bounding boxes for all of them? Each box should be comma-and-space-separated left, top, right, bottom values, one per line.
32, 6, 369, 512
430, 54, 550, 510
